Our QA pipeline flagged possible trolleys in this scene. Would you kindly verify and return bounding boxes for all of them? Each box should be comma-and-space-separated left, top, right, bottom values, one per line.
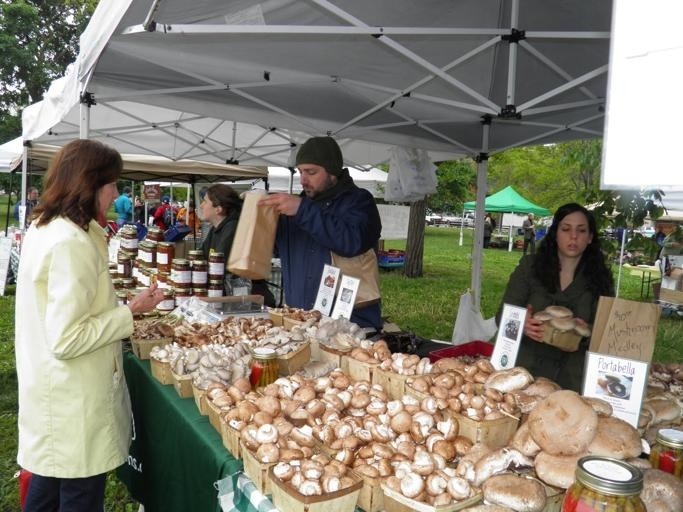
183, 230, 201, 247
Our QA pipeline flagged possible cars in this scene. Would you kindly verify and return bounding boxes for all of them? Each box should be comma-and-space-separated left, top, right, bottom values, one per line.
425, 210, 489, 227
601, 225, 657, 240
168, 200, 189, 210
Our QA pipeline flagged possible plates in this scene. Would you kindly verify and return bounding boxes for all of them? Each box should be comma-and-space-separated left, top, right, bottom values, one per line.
605, 382, 630, 399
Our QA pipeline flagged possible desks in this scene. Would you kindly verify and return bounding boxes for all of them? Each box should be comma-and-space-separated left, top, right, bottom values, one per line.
490, 234, 508, 247
99, 341, 366, 512
622, 263, 659, 297
10, 246, 20, 283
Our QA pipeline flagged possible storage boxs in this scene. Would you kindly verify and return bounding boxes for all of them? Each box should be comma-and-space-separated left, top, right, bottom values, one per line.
128, 306, 567, 512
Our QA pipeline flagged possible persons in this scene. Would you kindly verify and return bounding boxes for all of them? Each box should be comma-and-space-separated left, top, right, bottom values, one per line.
112, 188, 200, 236
192, 184, 278, 309
14, 137, 164, 511
495, 204, 615, 396
257, 137, 381, 338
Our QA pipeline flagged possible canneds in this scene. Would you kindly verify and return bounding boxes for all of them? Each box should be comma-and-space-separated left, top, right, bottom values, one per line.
106, 223, 226, 312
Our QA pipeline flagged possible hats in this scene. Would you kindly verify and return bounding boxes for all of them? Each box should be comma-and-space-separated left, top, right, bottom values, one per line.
295, 137, 343, 168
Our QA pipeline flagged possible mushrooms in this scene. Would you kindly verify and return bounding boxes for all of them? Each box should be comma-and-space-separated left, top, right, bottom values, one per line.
134, 302, 683, 512
